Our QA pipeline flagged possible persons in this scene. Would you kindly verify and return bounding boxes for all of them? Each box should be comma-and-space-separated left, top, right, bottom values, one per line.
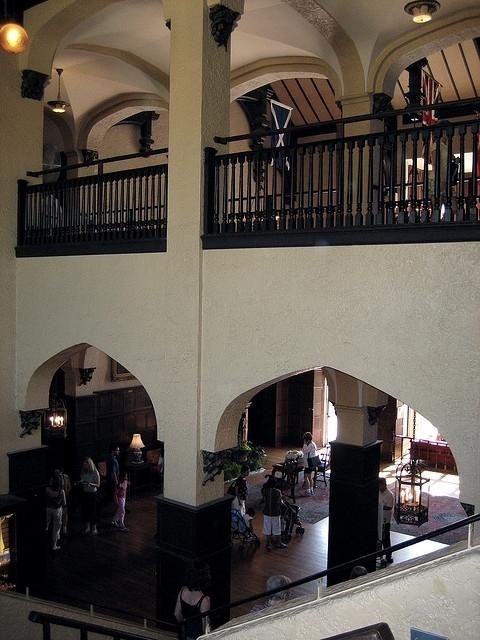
46, 465, 71, 550
227, 465, 288, 550
250, 575, 292, 613
44, 191, 62, 237
303, 432, 317, 496
110, 445, 131, 532
158, 443, 163, 492
379, 478, 394, 563
78, 457, 100, 535
174, 558, 212, 640
422, 120, 457, 221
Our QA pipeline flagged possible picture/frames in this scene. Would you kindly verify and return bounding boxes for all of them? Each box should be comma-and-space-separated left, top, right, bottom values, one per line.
111, 358, 139, 382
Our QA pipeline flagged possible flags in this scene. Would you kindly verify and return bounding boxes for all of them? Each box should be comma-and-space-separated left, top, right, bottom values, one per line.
271, 100, 293, 172
422, 70, 441, 126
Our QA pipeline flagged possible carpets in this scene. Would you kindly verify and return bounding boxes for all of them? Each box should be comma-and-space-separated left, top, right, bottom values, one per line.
224, 447, 329, 525
390, 487, 469, 546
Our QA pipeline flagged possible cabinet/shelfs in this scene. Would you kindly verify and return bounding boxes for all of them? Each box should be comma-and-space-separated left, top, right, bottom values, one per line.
76, 386, 157, 447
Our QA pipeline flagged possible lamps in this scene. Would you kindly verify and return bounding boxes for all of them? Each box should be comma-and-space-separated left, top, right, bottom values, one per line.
404, 0, 440, 24
130, 433, 147, 466
48, 393, 67, 440
0, 17, 28, 55
47, 69, 70, 114
366, 405, 431, 527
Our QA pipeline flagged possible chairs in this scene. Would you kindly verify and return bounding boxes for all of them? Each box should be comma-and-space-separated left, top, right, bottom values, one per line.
263, 446, 332, 503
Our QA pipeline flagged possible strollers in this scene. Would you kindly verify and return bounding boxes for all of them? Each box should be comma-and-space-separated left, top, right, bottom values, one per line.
280, 496, 306, 542
230, 507, 261, 555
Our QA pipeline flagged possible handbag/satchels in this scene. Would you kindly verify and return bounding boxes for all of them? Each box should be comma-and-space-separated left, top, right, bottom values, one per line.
307, 455, 321, 470
227, 486, 236, 496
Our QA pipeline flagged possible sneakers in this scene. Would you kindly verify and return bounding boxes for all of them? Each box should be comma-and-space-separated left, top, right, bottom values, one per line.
299, 490, 314, 497
112, 521, 129, 532
